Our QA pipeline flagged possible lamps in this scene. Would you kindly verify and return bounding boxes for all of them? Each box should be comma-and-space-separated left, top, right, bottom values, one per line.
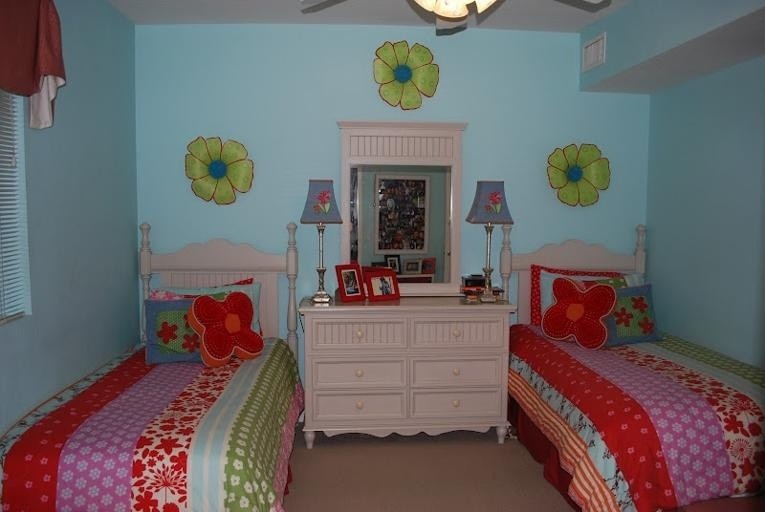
466, 177, 513, 303
415, 0, 498, 20
300, 179, 343, 303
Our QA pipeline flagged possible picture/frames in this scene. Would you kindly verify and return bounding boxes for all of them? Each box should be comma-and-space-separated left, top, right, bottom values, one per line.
362, 266, 392, 284
350, 166, 363, 264
351, 259, 358, 265
372, 262, 386, 267
423, 259, 435, 273
364, 271, 401, 302
333, 264, 363, 301
373, 173, 431, 255
385, 254, 401, 275
403, 261, 422, 274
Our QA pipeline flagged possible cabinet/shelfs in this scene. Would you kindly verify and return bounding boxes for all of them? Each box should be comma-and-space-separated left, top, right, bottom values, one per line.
297, 294, 519, 450
396, 274, 435, 283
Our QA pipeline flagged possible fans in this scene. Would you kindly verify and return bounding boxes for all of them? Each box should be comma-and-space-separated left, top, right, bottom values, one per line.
301, 0, 606, 31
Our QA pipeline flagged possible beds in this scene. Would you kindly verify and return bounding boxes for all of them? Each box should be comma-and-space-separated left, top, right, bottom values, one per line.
499, 218, 765, 512
1, 222, 303, 512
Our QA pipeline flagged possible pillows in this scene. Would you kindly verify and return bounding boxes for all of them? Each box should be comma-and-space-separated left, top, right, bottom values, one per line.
143, 295, 206, 364
186, 290, 265, 368
232, 276, 253, 286
149, 290, 211, 304
539, 268, 615, 320
164, 282, 261, 336
540, 275, 617, 350
529, 262, 621, 328
605, 284, 664, 346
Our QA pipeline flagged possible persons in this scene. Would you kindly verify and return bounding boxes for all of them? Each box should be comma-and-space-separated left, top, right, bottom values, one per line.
343, 272, 356, 293
378, 277, 390, 296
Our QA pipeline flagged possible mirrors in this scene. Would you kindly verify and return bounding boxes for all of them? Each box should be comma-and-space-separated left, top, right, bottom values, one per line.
337, 122, 463, 300
